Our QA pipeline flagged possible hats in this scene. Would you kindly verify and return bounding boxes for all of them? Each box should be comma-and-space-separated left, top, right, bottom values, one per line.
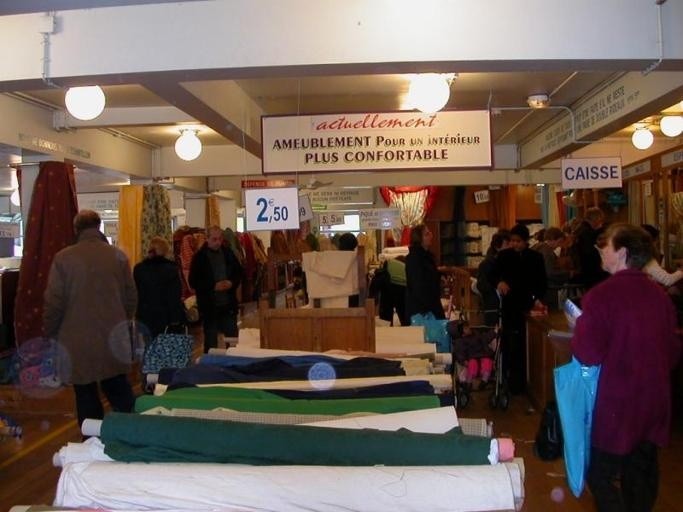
507, 222, 529, 239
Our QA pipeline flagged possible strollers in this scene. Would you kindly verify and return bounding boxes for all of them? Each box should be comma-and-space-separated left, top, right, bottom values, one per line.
445, 278, 510, 412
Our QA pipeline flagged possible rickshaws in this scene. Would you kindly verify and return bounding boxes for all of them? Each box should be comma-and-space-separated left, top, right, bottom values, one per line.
141, 322, 193, 395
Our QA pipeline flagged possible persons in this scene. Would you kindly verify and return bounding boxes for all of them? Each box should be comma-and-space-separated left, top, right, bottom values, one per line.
132, 236, 190, 392
39, 209, 137, 442
564, 216, 677, 511
187, 222, 245, 357
474, 221, 683, 397
454, 319, 499, 394
405, 223, 444, 326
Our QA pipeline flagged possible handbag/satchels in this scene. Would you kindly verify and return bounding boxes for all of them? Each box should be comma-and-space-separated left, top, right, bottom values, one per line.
531, 402, 564, 462
141, 333, 195, 375
550, 354, 602, 500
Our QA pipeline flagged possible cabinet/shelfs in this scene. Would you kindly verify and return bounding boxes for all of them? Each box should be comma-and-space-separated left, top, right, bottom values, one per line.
438, 219, 544, 275
